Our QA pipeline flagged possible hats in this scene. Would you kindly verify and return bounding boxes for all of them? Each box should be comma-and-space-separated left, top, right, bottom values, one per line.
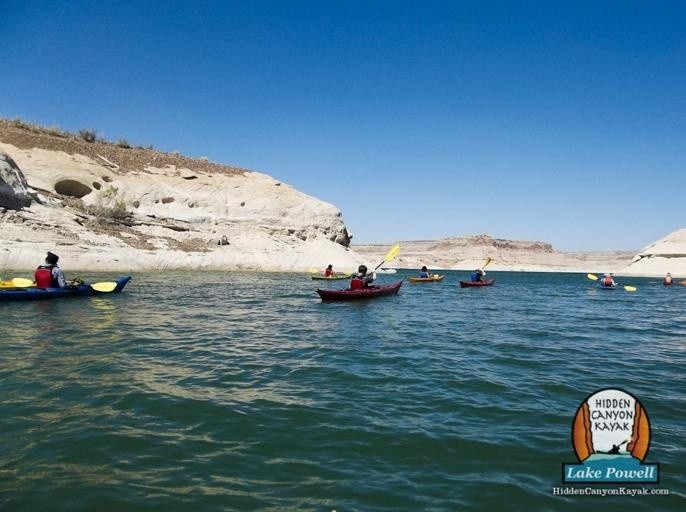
47, 252, 59, 264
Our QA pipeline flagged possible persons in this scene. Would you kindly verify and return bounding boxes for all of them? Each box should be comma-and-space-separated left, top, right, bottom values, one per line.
663, 272, 673, 285
420, 265, 432, 278
322, 265, 335, 278
600, 273, 616, 287
32, 251, 84, 290
348, 265, 384, 290
471, 268, 486, 283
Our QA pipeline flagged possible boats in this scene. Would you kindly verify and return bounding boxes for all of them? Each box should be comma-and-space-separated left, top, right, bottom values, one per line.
312, 275, 496, 299
599, 284, 617, 290
0, 275, 132, 300
664, 282, 672, 285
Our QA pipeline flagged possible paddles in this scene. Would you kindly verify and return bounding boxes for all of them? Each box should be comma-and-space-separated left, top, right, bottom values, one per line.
429, 273, 438, 279
482, 258, 491, 269
587, 274, 637, 292
309, 267, 351, 276
367, 245, 400, 277
11, 278, 117, 294
673, 280, 686, 286
